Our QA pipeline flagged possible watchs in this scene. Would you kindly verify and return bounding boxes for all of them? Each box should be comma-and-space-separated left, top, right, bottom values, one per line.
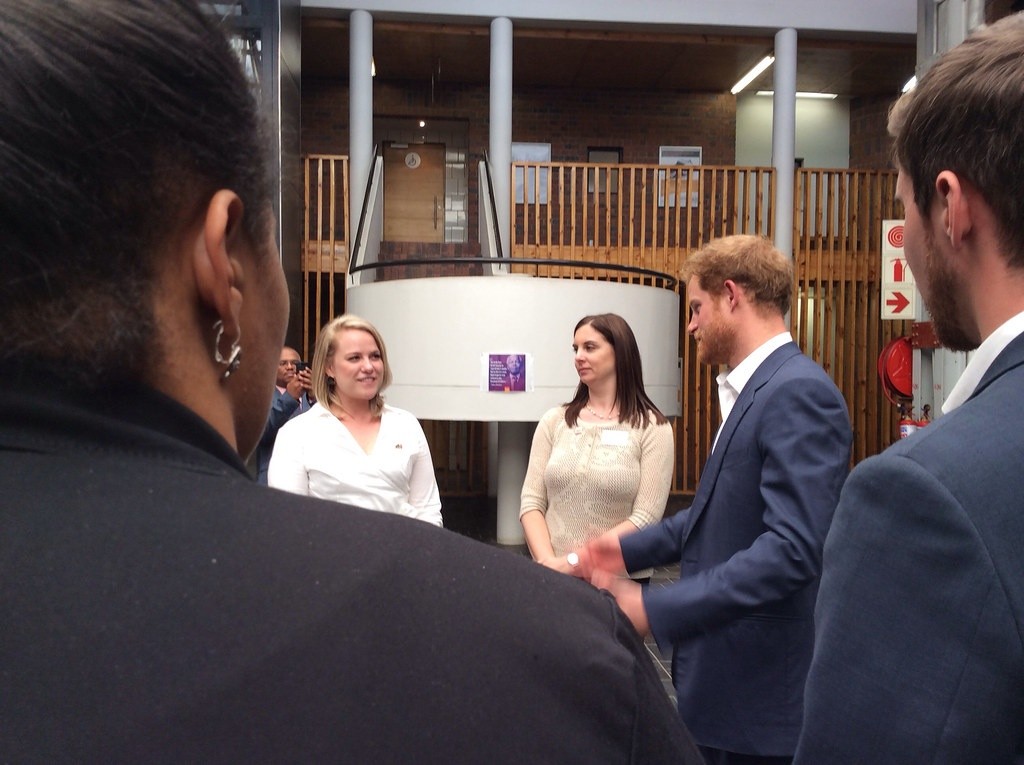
566, 552, 583, 579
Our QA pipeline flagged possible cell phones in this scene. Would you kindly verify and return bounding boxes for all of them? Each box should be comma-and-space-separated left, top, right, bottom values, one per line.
296, 362, 310, 375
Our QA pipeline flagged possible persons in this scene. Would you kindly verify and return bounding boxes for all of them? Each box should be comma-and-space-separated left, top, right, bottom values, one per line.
267, 314, 443, 528
0, 0, 712, 765
562, 234, 852, 765
262, 348, 313, 468
791, 8, 1024, 765
519, 313, 674, 662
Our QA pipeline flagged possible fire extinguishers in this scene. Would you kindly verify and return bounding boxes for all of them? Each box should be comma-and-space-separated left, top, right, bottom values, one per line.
900, 403, 931, 439
